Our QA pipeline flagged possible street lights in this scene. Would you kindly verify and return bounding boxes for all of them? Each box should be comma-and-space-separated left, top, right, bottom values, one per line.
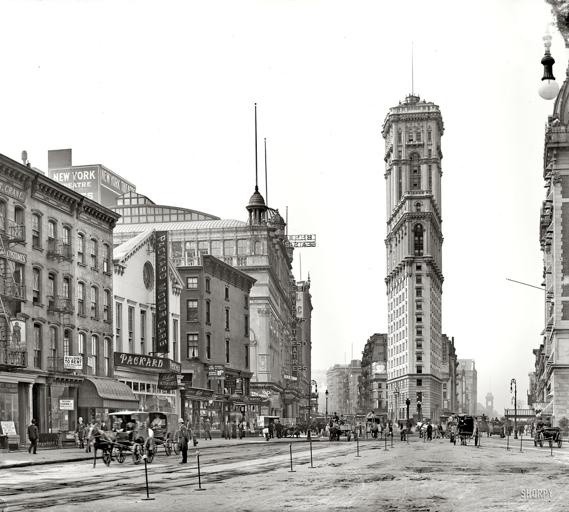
405, 397, 411, 429
306, 379, 319, 440
324, 389, 329, 427
508, 377, 520, 442
416, 400, 422, 423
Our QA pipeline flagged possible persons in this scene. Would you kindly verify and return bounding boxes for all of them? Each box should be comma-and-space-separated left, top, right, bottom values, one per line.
203, 417, 213, 441
27, 418, 40, 454
327, 410, 553, 442
220, 416, 327, 440
74, 414, 190, 464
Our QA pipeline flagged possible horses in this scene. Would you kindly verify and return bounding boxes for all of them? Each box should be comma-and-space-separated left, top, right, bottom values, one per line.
84, 421, 119, 466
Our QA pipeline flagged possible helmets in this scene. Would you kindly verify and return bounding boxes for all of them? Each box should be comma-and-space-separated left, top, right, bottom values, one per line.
178, 417, 185, 423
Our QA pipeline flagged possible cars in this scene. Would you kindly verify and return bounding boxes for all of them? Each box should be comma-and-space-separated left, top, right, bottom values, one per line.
532, 422, 563, 449
254, 415, 506, 437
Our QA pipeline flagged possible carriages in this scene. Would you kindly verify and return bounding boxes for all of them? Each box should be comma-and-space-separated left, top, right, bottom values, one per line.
84, 409, 182, 468
450, 413, 479, 447
